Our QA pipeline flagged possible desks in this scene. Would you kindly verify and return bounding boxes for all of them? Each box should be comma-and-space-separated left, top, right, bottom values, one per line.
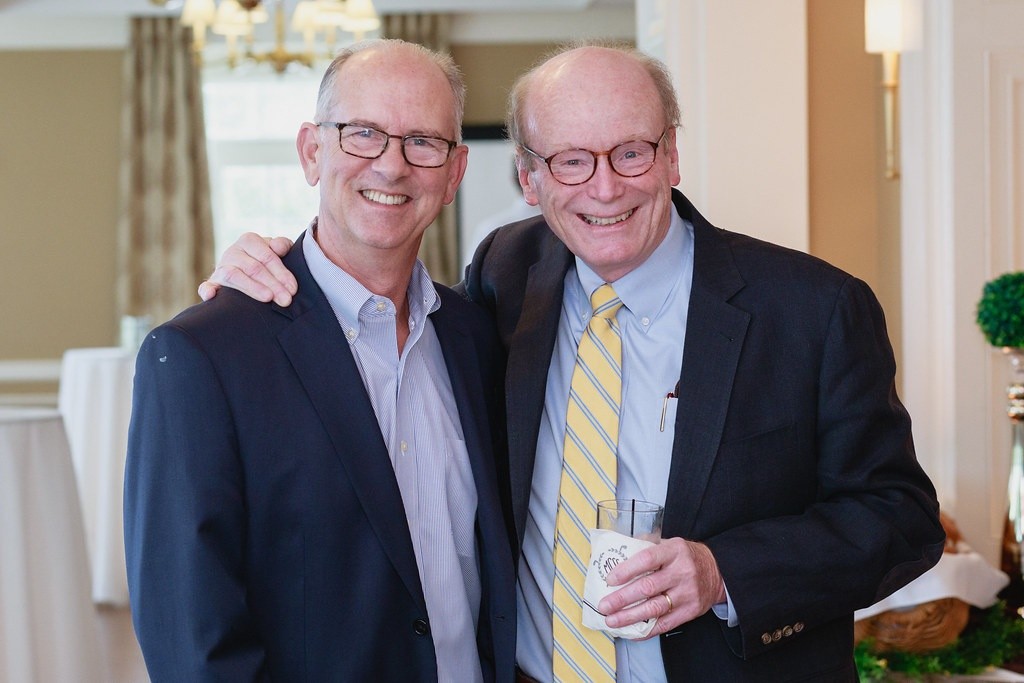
0, 407, 151, 683
59, 346, 140, 607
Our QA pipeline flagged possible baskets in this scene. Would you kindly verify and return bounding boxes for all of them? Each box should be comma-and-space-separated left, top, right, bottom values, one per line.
849, 511, 968, 651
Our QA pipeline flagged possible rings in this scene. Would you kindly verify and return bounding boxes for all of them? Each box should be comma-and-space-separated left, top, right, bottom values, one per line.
661, 592, 672, 612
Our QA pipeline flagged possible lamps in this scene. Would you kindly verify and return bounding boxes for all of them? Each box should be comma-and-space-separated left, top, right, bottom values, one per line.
182, 0, 381, 75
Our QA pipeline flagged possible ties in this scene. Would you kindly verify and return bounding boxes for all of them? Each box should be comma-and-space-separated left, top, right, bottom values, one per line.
552, 282, 623, 683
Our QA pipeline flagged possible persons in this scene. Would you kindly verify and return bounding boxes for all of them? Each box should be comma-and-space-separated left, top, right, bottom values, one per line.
197, 46, 946, 683
123, 38, 518, 683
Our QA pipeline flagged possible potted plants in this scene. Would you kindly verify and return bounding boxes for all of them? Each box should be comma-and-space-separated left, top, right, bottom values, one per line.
977, 270, 1023, 381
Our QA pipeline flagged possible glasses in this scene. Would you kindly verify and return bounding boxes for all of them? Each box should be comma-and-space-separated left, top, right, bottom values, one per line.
316, 121, 458, 168
521, 125, 672, 186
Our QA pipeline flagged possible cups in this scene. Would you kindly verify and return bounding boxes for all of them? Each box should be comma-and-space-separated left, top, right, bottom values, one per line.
596, 500, 664, 544
